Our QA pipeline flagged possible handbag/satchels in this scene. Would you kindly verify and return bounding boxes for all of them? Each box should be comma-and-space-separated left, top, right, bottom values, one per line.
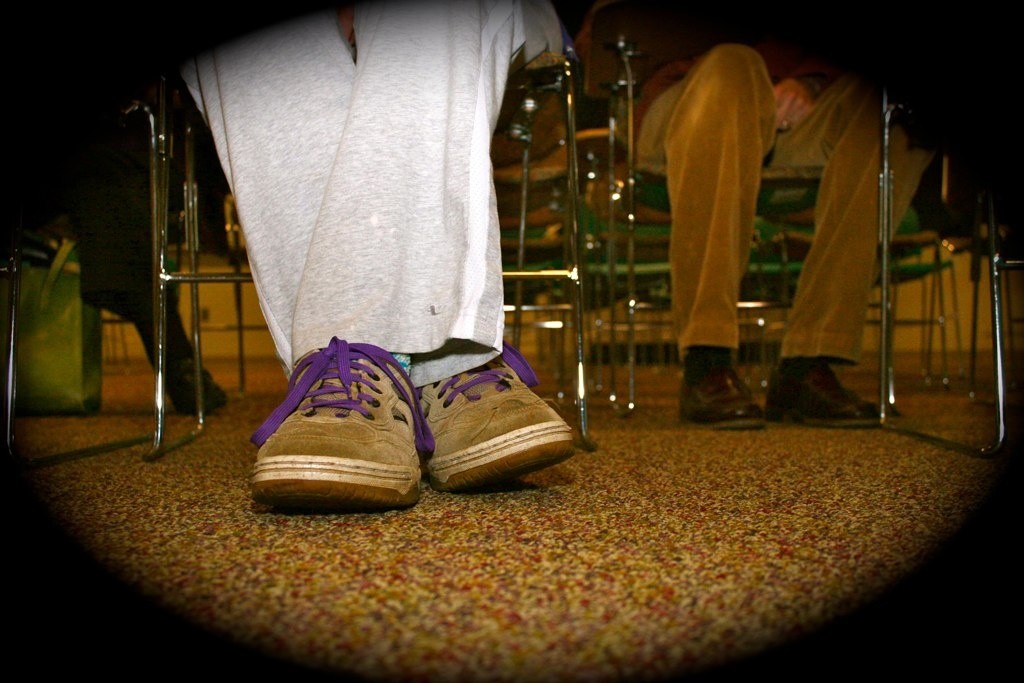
14, 241, 102, 416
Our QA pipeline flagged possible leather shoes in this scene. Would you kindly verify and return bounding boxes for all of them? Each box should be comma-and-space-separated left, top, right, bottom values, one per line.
679, 368, 764, 431
764, 364, 881, 425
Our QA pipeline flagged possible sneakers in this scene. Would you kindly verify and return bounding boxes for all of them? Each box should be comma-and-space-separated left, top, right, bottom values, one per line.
415, 340, 573, 492
247, 334, 436, 510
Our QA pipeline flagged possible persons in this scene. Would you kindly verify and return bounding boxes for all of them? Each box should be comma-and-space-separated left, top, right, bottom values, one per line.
910, 0, 1024, 261
172, 0, 578, 507
590, 1, 965, 430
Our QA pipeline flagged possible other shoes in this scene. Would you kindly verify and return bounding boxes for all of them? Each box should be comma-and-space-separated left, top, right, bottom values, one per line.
166, 365, 223, 415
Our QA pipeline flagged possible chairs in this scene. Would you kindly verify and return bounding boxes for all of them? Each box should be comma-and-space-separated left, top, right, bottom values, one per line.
136, 39, 1023, 452
